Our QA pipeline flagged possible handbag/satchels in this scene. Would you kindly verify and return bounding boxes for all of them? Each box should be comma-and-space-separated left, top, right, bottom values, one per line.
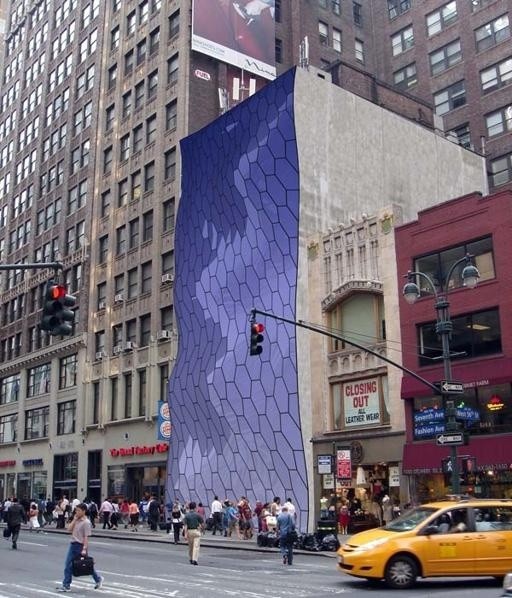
30, 512, 34, 516
72, 557, 94, 576
4, 526, 11, 537
287, 530, 298, 542
172, 511, 181, 518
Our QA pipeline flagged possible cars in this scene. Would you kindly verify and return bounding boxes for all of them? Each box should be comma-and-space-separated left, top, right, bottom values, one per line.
336, 498, 512, 588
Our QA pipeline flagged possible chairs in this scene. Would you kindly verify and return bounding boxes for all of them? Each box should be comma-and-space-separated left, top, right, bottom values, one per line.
438, 514, 452, 532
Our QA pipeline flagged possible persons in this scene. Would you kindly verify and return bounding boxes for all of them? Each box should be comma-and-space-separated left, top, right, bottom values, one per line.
55, 502, 105, 592
472, 507, 493, 532
1, 492, 296, 565
320, 478, 400, 534
447, 510, 466, 533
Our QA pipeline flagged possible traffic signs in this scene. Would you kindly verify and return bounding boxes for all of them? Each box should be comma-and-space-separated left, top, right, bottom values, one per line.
435, 432, 465, 446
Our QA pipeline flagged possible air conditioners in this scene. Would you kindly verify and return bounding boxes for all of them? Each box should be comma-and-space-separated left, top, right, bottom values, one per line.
160, 273, 174, 283
155, 329, 170, 339
98, 301, 105, 308
113, 344, 122, 354
124, 340, 133, 349
114, 294, 122, 302
94, 351, 104, 360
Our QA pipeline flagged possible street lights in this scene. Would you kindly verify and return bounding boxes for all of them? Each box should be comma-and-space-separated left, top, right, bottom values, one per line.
402, 253, 482, 496
440, 382, 464, 394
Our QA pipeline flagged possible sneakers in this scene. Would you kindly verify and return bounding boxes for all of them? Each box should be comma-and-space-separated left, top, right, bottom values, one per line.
56, 585, 71, 592
94, 576, 104, 589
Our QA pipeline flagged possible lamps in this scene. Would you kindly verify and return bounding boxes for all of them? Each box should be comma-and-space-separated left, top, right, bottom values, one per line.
486, 393, 505, 411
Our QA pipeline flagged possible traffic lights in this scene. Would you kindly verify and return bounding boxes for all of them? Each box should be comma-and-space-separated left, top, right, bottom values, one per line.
249, 323, 265, 356
441, 456, 454, 474
461, 457, 477, 475
39, 281, 67, 332
50, 292, 76, 336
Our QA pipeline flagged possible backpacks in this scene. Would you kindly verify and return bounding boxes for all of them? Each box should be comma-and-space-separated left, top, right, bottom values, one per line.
90, 502, 96, 515
